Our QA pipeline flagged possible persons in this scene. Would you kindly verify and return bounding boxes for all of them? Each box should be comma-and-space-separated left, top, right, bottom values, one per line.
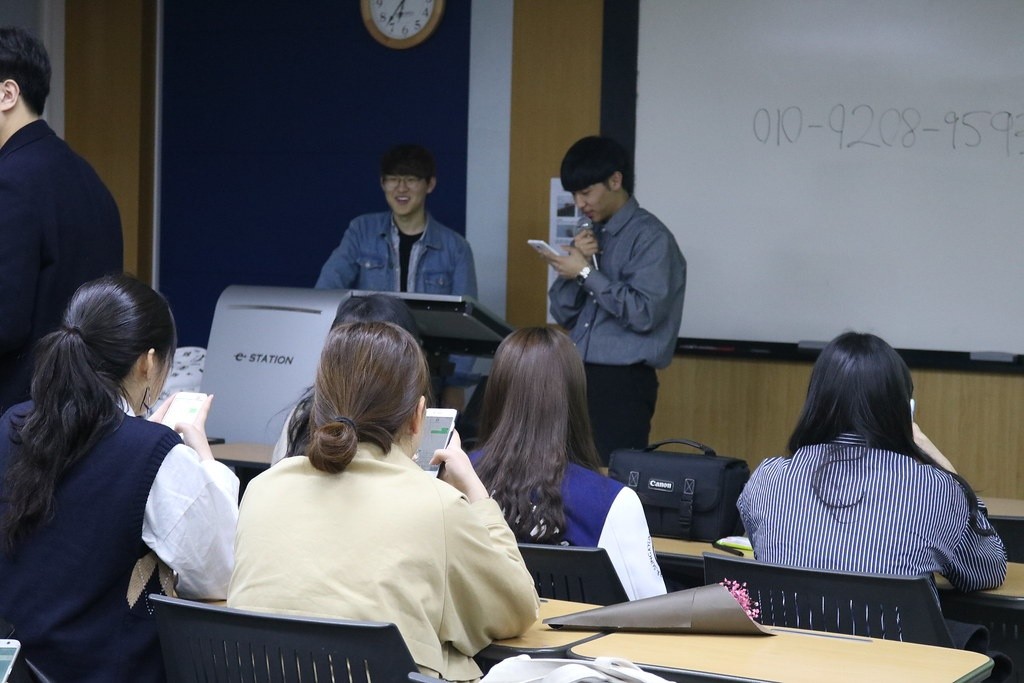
228, 318, 540, 683
0, 21, 124, 417
312, 143, 479, 298
539, 135, 688, 474
466, 326, 668, 601
736, 330, 1014, 683
0, 274, 240, 683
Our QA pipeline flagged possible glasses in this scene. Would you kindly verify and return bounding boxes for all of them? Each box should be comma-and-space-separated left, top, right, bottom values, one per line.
382, 173, 421, 187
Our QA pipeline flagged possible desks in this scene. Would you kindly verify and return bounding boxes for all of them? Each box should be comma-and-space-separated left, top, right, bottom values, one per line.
207, 443, 276, 509
651, 536, 755, 594
478, 593, 609, 661
933, 560, 1024, 683
565, 624, 996, 683
979, 497, 1024, 564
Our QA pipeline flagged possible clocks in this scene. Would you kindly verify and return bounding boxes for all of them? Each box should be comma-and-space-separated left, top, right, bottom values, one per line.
360, 0, 446, 50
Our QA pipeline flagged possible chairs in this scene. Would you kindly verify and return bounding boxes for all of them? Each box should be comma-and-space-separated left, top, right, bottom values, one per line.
516, 543, 630, 605
702, 551, 955, 649
148, 593, 421, 683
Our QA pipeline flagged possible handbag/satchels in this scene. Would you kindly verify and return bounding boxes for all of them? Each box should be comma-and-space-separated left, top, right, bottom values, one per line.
606, 438, 751, 545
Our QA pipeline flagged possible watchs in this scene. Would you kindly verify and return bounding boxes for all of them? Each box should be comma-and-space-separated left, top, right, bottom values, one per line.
576, 264, 593, 286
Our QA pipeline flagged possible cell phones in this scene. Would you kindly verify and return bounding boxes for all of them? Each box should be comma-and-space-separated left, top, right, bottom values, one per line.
411, 408, 457, 478
528, 240, 560, 257
162, 392, 208, 441
